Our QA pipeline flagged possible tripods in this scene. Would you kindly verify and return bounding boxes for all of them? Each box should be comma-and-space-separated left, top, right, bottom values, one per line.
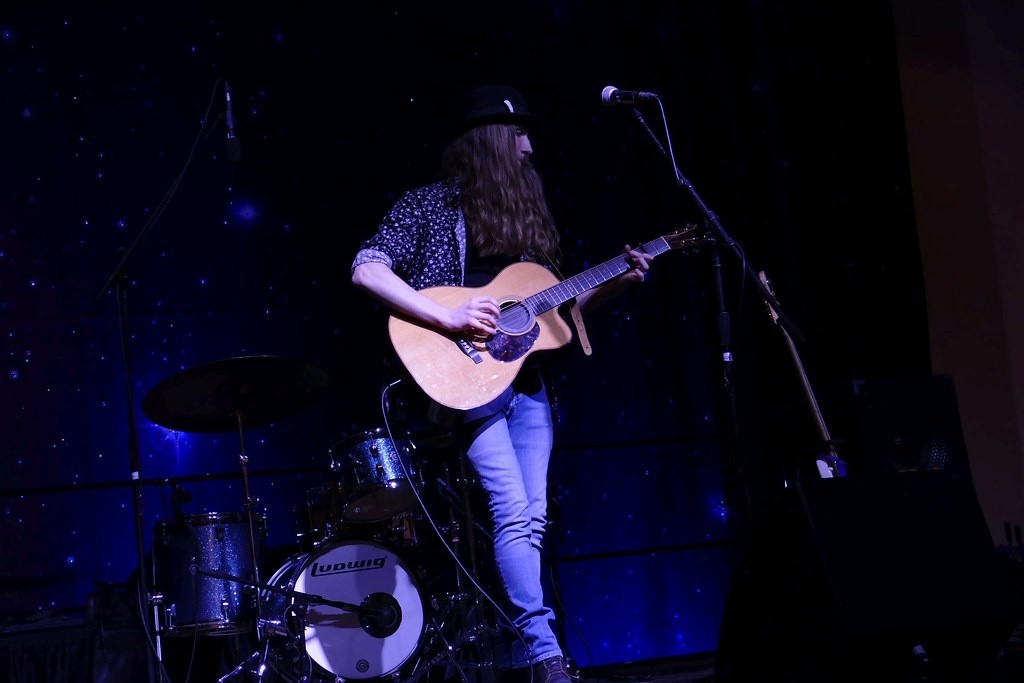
408, 429, 502, 683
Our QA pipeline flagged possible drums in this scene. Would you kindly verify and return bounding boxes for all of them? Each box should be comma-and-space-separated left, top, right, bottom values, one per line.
302, 495, 420, 552
326, 426, 427, 525
255, 540, 430, 683
151, 512, 269, 638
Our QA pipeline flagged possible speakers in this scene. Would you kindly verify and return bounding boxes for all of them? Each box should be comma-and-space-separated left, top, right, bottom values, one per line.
715, 375, 1024, 683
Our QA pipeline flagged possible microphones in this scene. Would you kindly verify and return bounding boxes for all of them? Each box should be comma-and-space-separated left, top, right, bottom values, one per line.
225, 81, 243, 161
602, 86, 659, 104
376, 607, 397, 627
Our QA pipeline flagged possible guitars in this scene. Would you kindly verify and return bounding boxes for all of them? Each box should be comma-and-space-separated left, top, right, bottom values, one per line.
388, 221, 717, 427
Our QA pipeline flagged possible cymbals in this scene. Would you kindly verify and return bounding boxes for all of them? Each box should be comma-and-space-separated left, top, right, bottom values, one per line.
144, 354, 334, 435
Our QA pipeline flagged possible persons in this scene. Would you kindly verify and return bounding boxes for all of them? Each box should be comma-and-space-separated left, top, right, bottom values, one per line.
350, 87, 654, 683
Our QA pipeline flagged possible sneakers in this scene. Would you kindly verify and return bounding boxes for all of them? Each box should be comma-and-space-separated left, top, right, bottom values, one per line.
535, 655, 577, 682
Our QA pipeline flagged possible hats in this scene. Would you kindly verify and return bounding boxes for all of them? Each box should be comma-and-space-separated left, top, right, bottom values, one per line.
452, 84, 534, 128
